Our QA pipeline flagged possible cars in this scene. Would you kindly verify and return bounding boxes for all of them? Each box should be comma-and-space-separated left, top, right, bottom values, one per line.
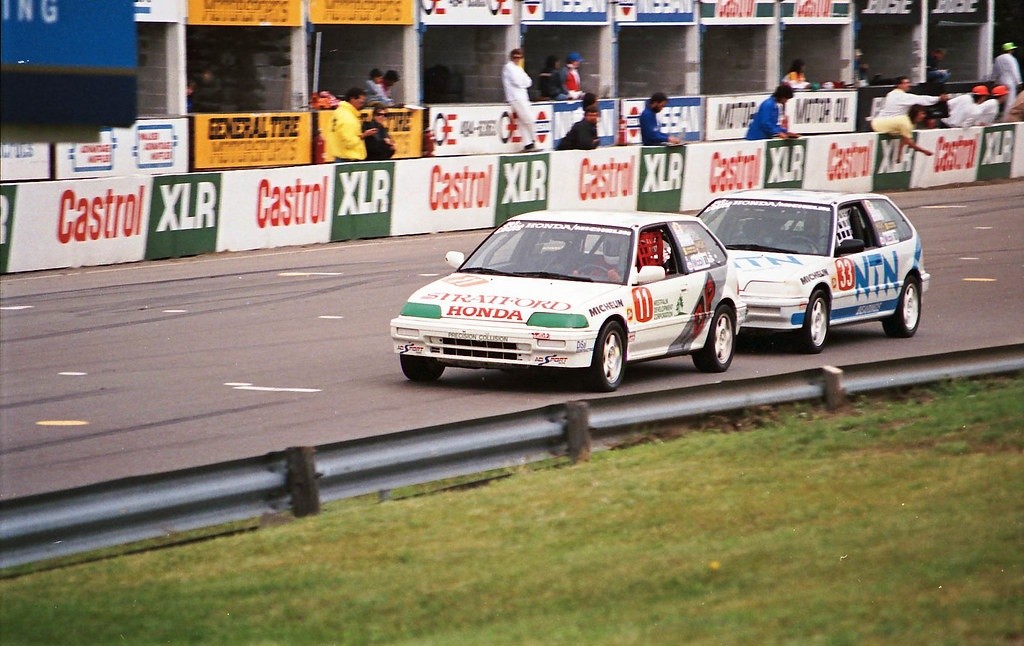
689, 187, 930, 353
389, 209, 749, 393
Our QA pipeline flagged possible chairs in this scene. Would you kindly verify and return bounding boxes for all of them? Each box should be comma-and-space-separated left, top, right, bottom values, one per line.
742, 219, 774, 240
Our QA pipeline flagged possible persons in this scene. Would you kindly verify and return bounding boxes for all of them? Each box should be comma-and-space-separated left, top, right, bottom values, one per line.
328, 87, 379, 162
868, 42, 1024, 164
362, 104, 397, 161
501, 48, 544, 152
854, 47, 871, 87
362, 69, 406, 108
781, 59, 812, 89
571, 234, 624, 282
538, 50, 585, 100
555, 91, 601, 151
746, 85, 798, 141
639, 92, 681, 145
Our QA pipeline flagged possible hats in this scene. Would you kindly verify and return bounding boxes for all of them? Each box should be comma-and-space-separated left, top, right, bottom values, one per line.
1001, 42, 1018, 52
566, 51, 585, 61
991, 85, 1009, 96
971, 85, 990, 95
511, 49, 523, 59
